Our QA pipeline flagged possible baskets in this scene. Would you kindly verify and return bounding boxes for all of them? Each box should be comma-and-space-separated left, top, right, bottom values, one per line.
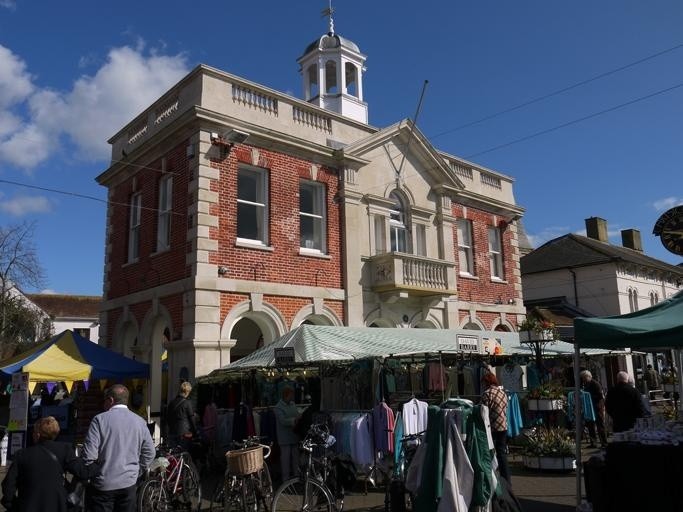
226, 446, 264, 475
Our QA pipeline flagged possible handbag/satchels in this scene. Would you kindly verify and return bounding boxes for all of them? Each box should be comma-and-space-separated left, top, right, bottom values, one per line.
62, 473, 80, 506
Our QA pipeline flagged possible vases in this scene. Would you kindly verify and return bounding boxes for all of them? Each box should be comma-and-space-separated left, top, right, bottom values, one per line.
527, 398, 564, 411
517, 329, 554, 343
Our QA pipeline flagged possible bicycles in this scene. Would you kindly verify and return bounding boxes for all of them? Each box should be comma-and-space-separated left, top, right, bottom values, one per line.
208, 434, 274, 512
383, 430, 428, 510
270, 416, 351, 512
135, 434, 205, 512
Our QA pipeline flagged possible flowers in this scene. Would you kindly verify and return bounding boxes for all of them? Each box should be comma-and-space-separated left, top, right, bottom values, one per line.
525, 380, 569, 416
516, 317, 561, 346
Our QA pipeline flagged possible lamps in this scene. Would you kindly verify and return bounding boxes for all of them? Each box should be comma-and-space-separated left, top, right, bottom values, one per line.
501, 210, 524, 227
216, 126, 250, 152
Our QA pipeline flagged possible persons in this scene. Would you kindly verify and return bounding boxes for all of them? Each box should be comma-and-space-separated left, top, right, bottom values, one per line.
479, 374, 512, 488
274, 386, 309, 484
0, 382, 197, 512
580, 364, 658, 449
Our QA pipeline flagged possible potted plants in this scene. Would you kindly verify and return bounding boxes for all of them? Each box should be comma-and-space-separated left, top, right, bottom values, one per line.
519, 422, 576, 471
